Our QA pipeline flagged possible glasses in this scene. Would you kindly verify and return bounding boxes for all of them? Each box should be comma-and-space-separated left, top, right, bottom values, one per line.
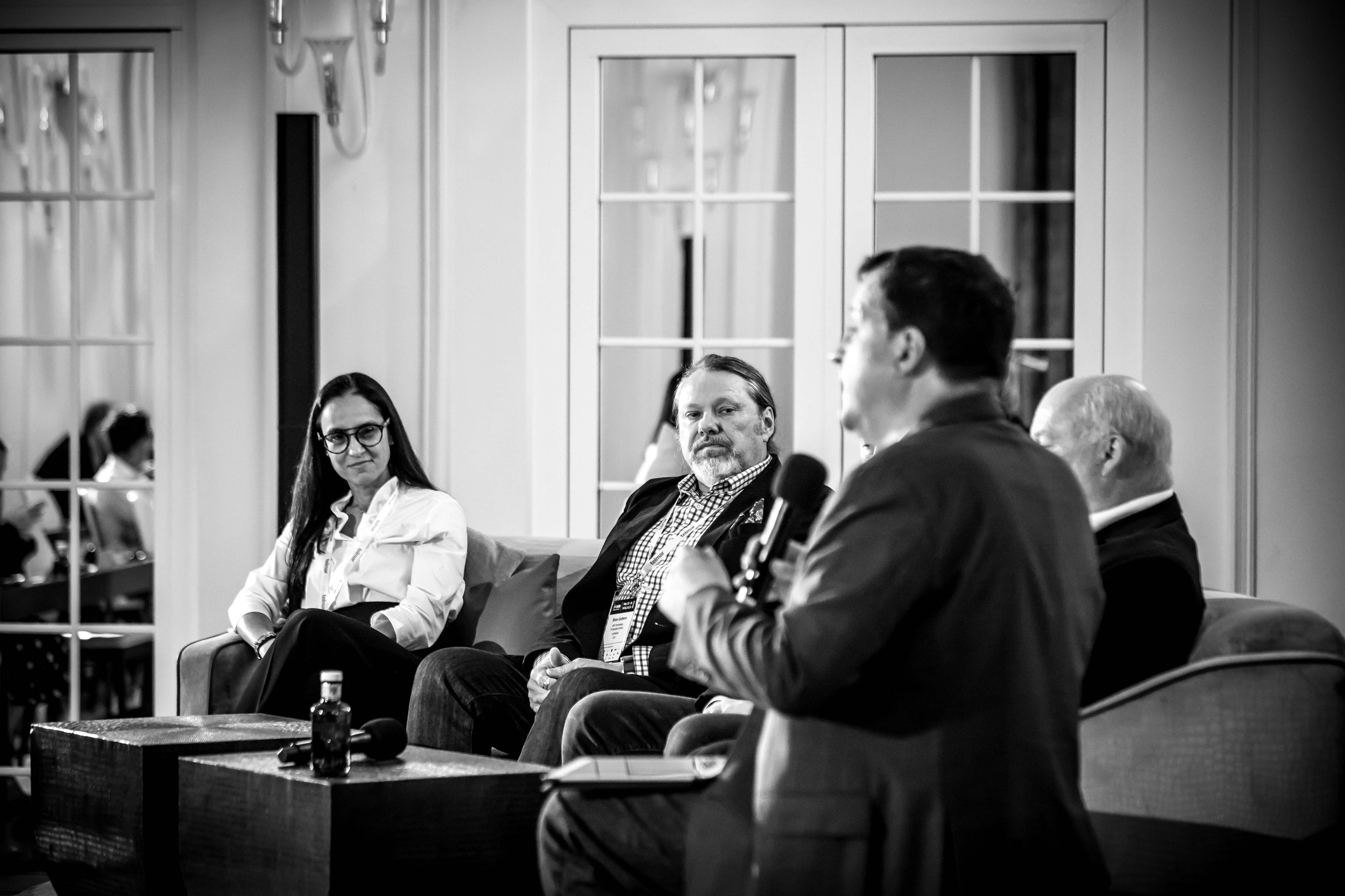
317, 418, 392, 454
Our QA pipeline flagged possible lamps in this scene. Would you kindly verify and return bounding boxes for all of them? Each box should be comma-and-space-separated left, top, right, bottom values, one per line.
264, 0, 396, 158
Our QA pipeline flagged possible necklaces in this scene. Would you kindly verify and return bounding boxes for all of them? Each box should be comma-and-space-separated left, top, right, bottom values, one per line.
351, 500, 365, 514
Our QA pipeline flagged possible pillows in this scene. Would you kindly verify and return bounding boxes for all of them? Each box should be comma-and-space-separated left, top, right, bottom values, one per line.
480, 558, 567, 659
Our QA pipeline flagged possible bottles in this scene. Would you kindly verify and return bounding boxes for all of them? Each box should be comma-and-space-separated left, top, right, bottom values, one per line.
311, 670, 351, 775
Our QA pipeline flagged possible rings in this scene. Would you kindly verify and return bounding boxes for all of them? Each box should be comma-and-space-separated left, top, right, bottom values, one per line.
538, 676, 550, 690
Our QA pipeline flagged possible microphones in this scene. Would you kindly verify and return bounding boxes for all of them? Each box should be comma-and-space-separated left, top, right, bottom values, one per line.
276, 718, 408, 767
746, 453, 827, 596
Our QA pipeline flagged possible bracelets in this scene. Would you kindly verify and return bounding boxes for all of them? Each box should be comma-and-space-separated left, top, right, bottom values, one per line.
254, 631, 278, 660
608, 662, 613, 671
620, 656, 635, 675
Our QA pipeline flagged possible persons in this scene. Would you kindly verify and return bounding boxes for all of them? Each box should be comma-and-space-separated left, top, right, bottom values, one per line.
635, 365, 689, 489
407, 355, 832, 766
1029, 372, 1205, 710
536, 246, 1108, 896
0, 398, 153, 625
229, 372, 468, 728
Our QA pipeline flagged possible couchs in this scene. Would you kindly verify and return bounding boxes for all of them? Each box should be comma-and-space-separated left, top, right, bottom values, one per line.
177, 526, 1345, 895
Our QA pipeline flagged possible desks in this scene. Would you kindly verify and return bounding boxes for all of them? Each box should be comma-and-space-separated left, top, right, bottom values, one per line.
27, 712, 362, 896
178, 746, 554, 896
